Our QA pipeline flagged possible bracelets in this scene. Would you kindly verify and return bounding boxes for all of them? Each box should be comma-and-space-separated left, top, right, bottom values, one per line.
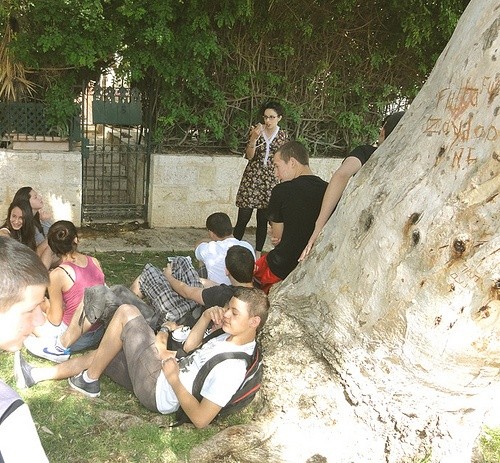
247, 141, 256, 148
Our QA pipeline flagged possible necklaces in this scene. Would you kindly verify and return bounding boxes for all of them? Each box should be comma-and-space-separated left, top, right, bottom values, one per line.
255, 129, 276, 148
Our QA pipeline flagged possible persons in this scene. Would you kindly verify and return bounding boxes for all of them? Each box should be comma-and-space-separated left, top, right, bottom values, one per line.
195, 212, 256, 286
297, 111, 406, 262
129, 245, 255, 323
0, 186, 61, 272
252, 140, 342, 295
0, 235, 51, 463
233, 101, 290, 259
32, 220, 104, 353
23, 284, 228, 363
13, 287, 270, 429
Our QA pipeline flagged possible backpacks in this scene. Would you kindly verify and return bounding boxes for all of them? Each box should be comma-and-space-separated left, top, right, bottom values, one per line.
159, 328, 264, 430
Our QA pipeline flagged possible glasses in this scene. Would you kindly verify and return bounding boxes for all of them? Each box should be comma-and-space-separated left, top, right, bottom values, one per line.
262, 116, 276, 120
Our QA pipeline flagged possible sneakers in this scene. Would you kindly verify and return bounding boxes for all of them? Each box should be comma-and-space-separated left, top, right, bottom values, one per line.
67, 368, 101, 398
26, 335, 71, 363
14, 350, 35, 388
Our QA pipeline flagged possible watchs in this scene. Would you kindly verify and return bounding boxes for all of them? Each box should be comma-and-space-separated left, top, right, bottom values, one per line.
160, 326, 170, 334
